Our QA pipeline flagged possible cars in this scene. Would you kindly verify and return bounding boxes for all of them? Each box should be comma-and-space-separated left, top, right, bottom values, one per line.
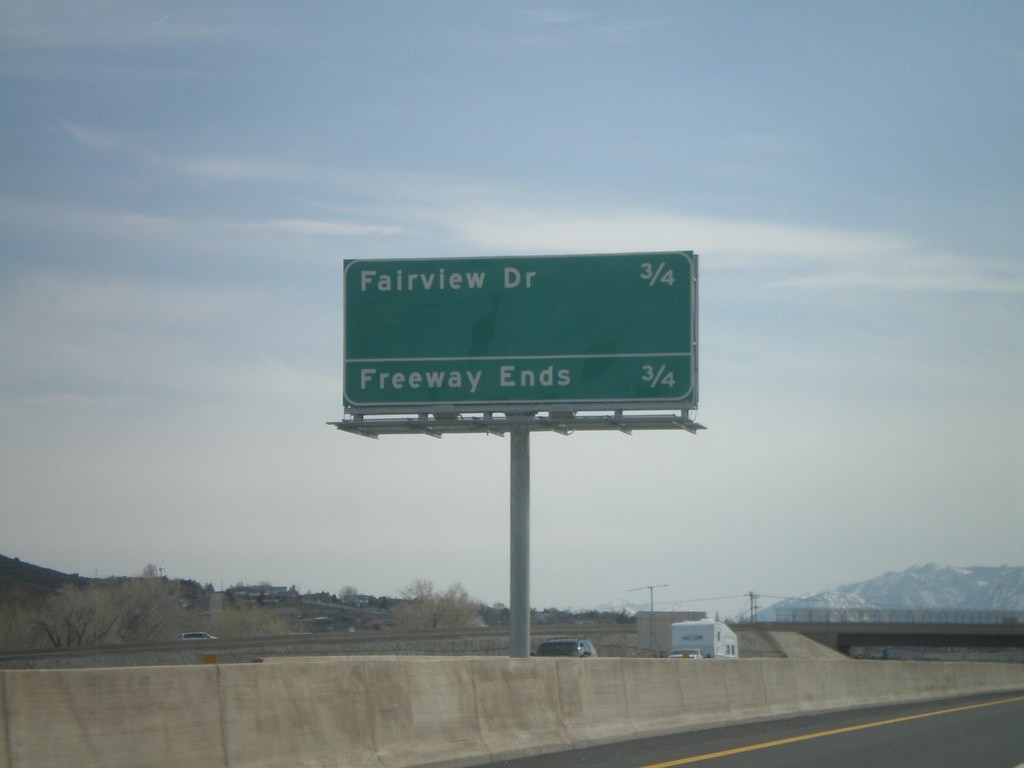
667, 649, 705, 659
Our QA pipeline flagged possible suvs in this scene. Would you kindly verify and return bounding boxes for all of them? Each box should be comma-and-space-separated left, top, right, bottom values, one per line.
178, 632, 218, 639
530, 637, 598, 659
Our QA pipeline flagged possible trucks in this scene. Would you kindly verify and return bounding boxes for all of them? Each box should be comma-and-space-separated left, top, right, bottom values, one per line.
671, 619, 739, 659
